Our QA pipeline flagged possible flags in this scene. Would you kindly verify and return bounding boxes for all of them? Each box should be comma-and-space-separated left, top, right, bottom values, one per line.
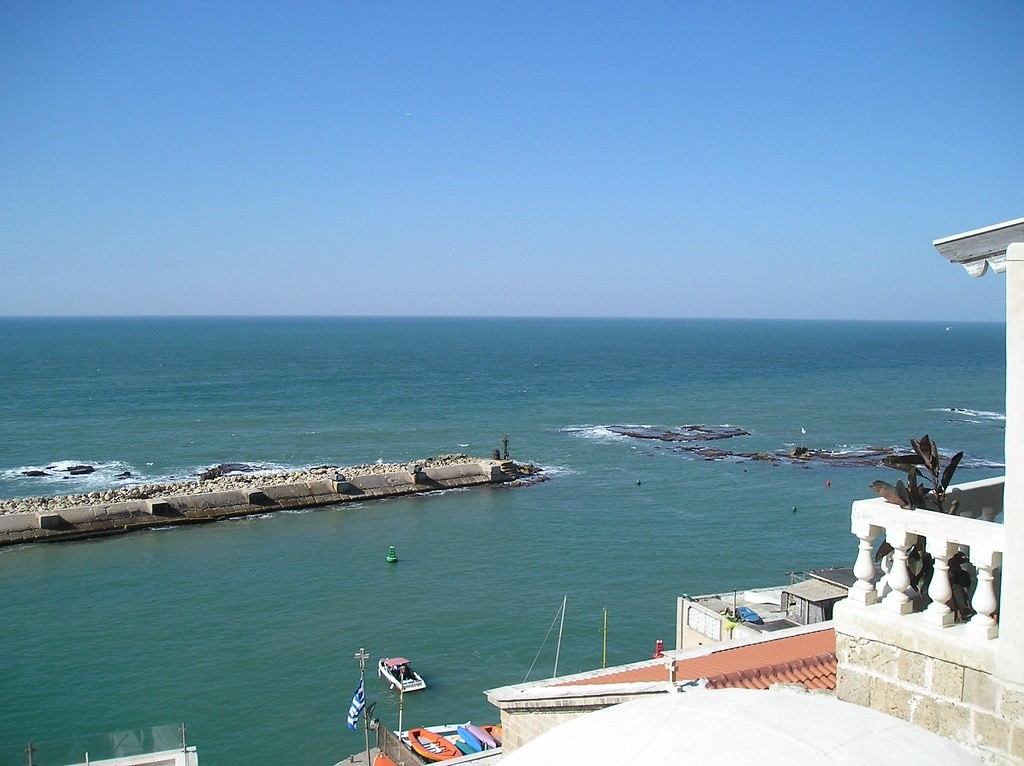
346, 677, 364, 733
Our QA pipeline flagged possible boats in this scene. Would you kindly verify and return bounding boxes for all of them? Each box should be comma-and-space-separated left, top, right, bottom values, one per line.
408, 725, 462, 762
455, 721, 498, 756
378, 656, 426, 694
736, 606, 760, 621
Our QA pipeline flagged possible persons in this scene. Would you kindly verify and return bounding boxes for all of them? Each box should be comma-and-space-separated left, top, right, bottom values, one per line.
725, 607, 742, 622
391, 668, 400, 679
